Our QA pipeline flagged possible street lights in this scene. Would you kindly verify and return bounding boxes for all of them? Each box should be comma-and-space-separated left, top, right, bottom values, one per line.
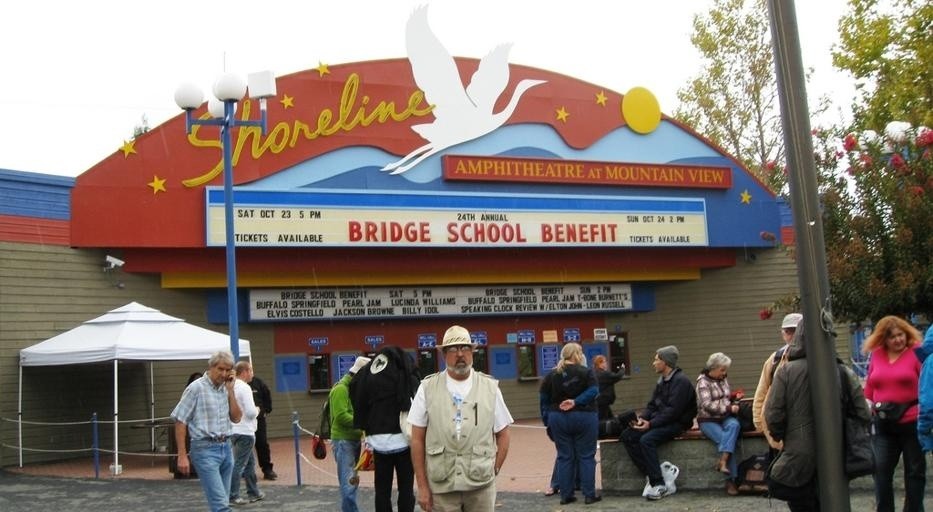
174, 72, 279, 362
843, 118, 931, 167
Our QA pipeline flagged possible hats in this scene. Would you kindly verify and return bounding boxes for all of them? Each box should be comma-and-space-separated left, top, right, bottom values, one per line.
781, 313, 803, 328
435, 325, 477, 351
657, 345, 679, 368
349, 356, 372, 374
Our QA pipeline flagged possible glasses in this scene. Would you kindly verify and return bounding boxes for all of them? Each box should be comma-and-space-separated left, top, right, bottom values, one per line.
447, 346, 470, 352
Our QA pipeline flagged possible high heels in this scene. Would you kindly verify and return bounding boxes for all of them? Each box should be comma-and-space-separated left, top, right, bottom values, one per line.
715, 458, 730, 475
725, 481, 739, 496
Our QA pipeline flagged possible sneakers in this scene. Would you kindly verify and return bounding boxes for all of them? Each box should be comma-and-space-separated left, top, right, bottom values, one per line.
229, 498, 248, 505
250, 492, 265, 502
647, 486, 670, 499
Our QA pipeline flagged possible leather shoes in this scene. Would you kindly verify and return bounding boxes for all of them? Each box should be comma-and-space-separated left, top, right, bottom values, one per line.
584, 494, 601, 503
560, 498, 577, 504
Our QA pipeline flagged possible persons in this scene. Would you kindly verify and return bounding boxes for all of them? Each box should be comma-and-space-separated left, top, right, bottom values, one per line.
349, 345, 421, 511
695, 352, 744, 494
620, 345, 697, 500
859, 316, 933, 511
753, 313, 872, 511
246, 377, 278, 480
186, 373, 202, 386
407, 324, 514, 512
327, 356, 372, 511
171, 351, 243, 512
229, 360, 267, 505
540, 343, 626, 504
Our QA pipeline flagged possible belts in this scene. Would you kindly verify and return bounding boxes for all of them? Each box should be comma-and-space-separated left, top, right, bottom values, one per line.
206, 435, 229, 442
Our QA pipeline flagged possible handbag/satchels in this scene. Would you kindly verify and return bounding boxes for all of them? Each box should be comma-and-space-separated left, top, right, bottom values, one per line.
312, 437, 326, 459
353, 450, 376, 471
737, 401, 752, 431
873, 400, 903, 420
642, 462, 680, 497
764, 461, 816, 500
843, 416, 875, 477
318, 398, 330, 439
